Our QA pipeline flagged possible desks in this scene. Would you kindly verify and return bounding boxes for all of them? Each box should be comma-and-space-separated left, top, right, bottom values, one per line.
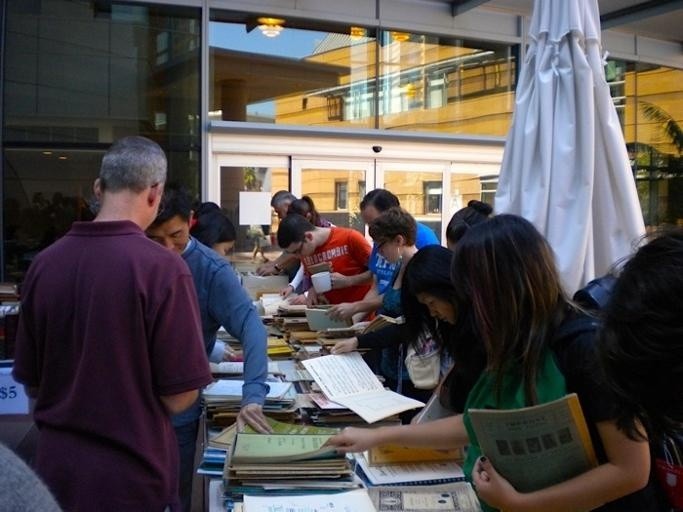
208, 263, 487, 512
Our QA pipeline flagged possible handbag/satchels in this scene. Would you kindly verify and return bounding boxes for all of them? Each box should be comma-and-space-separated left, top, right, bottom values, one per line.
404, 337, 442, 390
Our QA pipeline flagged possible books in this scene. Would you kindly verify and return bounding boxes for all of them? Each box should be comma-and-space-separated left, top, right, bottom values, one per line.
197, 275, 482, 511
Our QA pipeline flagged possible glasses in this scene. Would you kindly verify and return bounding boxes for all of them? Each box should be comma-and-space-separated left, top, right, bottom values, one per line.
150, 182, 167, 217
292, 240, 304, 255
373, 240, 386, 253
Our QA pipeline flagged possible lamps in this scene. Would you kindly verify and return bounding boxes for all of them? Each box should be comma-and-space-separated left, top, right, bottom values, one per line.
258, 16, 285, 36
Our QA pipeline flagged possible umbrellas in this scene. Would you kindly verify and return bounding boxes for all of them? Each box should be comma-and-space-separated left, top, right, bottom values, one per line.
491, 0, 649, 302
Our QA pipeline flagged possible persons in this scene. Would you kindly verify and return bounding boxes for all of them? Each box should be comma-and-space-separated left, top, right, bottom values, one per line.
1, 135, 270, 511
323, 191, 681, 511
255, 192, 374, 308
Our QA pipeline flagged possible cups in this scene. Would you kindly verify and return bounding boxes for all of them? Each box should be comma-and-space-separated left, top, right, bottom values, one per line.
311, 271, 333, 294
